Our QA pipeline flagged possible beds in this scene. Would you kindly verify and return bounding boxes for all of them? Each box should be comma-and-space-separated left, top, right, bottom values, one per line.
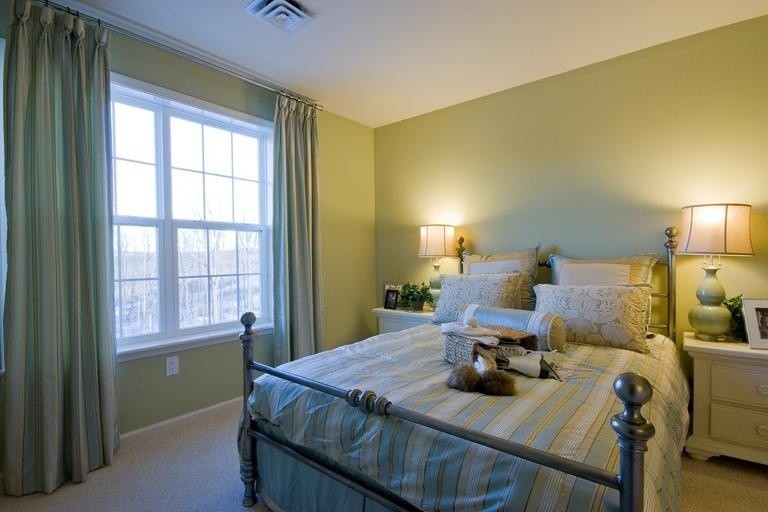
236, 227, 679, 510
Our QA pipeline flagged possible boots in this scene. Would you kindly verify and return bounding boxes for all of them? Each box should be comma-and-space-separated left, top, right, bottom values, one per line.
488, 345, 550, 379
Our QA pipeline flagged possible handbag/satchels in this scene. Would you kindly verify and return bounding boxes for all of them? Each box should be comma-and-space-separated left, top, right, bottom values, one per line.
443, 325, 538, 369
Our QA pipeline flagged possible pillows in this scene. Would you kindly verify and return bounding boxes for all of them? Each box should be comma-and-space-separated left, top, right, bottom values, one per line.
430, 242, 659, 354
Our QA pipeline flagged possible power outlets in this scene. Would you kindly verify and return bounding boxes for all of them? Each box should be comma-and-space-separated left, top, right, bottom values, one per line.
165, 355, 180, 378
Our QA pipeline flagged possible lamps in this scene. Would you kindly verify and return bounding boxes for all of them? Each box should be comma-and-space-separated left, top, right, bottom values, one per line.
672, 201, 754, 342
415, 224, 458, 312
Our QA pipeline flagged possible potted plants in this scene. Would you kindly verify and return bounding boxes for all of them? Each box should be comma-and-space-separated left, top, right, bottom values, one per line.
397, 282, 434, 312
721, 293, 747, 343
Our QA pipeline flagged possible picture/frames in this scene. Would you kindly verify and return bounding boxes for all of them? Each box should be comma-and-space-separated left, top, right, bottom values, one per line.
742, 299, 767, 350
383, 289, 398, 310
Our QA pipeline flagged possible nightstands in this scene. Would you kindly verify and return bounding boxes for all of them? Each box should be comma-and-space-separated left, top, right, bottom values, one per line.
369, 307, 434, 335
681, 331, 767, 466
